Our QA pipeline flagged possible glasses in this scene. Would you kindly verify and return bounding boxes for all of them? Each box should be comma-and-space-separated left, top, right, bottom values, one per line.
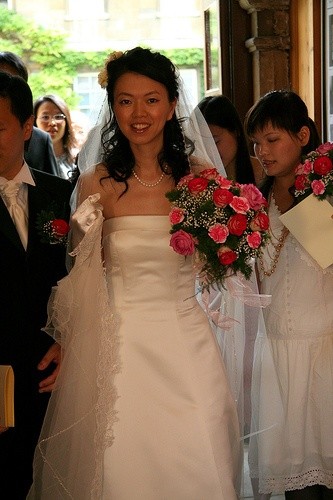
37, 114, 66, 124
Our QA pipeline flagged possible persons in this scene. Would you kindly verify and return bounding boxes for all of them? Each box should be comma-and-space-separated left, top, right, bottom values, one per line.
33, 94, 81, 179
26, 46, 285, 500
0, 51, 63, 176
188, 95, 268, 197
0, 70, 78, 500
248, 89, 333, 500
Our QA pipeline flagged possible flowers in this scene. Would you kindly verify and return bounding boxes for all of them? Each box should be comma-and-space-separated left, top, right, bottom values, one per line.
166, 169, 270, 299
97, 51, 123, 90
288, 142, 333, 205
43, 218, 70, 245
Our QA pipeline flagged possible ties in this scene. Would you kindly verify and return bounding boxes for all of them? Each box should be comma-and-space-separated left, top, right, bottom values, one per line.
2, 180, 28, 251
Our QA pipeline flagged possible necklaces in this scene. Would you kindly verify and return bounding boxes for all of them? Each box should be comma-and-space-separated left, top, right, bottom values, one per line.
259, 221, 291, 278
131, 165, 168, 187
